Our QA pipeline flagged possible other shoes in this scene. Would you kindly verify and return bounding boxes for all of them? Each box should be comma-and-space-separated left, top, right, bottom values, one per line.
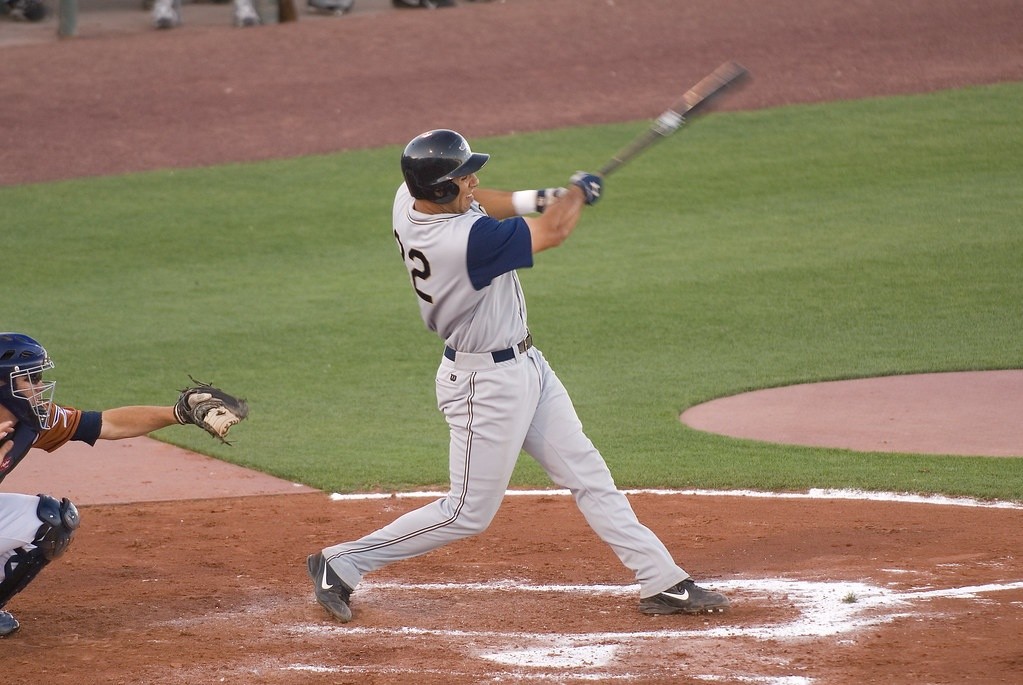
1, 611, 20, 637
232, 4, 258, 27
154, 7, 176, 27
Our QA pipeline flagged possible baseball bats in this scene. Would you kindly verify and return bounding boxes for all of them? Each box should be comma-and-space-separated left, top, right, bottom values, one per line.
595, 60, 755, 179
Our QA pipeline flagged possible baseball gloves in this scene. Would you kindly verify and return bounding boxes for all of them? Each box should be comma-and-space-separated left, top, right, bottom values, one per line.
172, 373, 251, 447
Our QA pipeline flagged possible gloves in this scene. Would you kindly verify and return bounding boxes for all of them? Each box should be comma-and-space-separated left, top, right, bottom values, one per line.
571, 169, 602, 204
537, 188, 568, 213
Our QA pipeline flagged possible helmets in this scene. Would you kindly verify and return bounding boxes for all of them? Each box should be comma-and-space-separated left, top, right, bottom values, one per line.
401, 129, 490, 203
0, 332, 55, 431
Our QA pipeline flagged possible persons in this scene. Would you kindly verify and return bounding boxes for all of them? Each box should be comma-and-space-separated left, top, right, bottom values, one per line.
306, 129, 728, 622
0, 332, 247, 637
153, 0, 455, 29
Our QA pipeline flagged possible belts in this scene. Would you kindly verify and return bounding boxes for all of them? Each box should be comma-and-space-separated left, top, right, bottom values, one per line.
444, 334, 533, 363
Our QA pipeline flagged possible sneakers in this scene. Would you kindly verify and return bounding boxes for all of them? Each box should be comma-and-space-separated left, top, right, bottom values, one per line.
307, 550, 354, 622
640, 579, 728, 617
